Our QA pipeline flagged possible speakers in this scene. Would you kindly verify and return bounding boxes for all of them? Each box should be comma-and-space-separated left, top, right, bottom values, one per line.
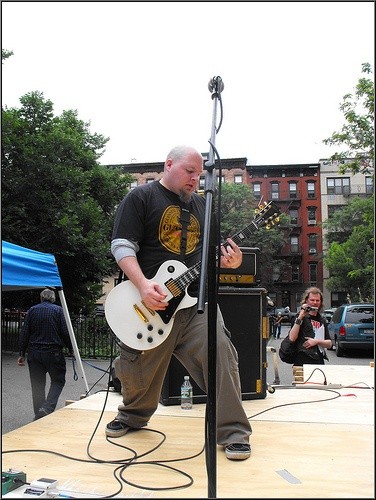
160, 286, 267, 406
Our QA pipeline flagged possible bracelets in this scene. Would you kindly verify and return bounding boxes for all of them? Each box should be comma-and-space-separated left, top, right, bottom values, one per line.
294, 318, 303, 326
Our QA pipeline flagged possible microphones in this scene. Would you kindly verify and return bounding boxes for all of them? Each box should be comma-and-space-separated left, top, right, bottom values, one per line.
208, 77, 225, 93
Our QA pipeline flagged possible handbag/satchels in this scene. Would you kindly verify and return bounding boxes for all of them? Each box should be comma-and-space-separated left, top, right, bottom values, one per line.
279, 313, 305, 364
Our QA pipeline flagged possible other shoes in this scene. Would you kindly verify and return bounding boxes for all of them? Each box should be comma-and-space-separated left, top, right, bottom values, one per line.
35, 411, 47, 421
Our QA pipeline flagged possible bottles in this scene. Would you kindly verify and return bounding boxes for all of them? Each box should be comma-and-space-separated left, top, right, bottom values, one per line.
181, 376, 193, 410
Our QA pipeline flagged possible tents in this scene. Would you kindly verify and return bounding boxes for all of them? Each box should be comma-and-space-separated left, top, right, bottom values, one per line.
2, 240, 90, 397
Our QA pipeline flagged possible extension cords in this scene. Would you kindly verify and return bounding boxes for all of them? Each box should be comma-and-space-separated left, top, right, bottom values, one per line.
296, 383, 342, 388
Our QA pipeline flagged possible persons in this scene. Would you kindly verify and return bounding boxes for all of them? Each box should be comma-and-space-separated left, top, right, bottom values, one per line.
16, 288, 75, 421
102, 143, 253, 460
273, 312, 284, 338
289, 287, 333, 365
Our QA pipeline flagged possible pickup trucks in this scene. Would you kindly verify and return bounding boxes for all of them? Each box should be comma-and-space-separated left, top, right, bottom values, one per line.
267, 309, 298, 323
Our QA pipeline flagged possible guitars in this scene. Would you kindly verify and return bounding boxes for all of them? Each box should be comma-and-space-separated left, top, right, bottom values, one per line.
104, 202, 284, 356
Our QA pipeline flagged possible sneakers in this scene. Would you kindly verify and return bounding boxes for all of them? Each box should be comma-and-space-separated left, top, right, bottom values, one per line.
225, 444, 251, 460
105, 419, 132, 438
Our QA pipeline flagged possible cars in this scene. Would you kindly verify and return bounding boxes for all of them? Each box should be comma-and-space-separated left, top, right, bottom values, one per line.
322, 304, 374, 357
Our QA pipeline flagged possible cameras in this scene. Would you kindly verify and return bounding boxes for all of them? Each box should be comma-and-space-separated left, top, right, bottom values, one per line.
305, 306, 319, 312
